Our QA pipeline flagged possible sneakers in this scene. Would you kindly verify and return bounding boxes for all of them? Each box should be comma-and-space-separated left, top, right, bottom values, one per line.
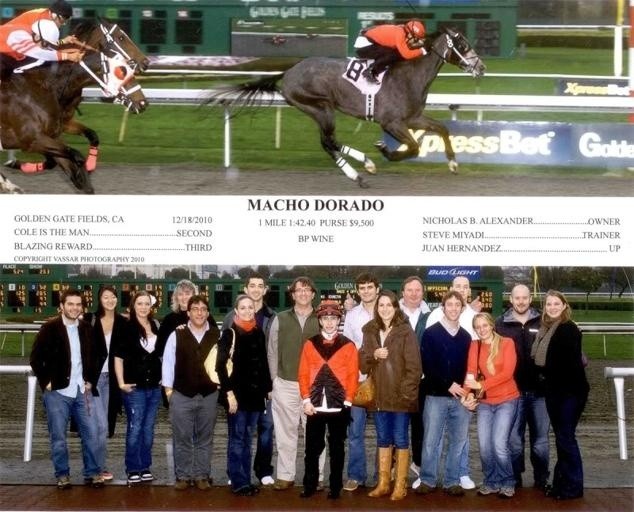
260, 476, 275, 485
193, 479, 212, 492
298, 480, 325, 500
533, 480, 585, 502
126, 469, 154, 484
83, 472, 114, 486
442, 484, 466, 497
459, 474, 476, 491
172, 479, 190, 491
474, 482, 500, 498
342, 475, 363, 493
233, 485, 260, 497
275, 478, 296, 492
326, 490, 342, 501
361, 66, 382, 86
498, 485, 517, 499
407, 460, 437, 496
55, 477, 72, 491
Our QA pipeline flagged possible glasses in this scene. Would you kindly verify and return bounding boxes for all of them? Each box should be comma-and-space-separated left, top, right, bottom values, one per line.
294, 288, 314, 293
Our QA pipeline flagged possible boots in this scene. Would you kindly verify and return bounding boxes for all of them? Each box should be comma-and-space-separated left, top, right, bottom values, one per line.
390, 447, 411, 502
367, 445, 395, 499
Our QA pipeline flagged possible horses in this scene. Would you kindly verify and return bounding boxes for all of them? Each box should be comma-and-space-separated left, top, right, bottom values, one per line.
2, 16, 150, 175
194, 24, 486, 189
0, 42, 149, 194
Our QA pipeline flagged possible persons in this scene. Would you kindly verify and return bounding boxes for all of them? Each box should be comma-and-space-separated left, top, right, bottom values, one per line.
1, 19, 82, 62
352, 21, 427, 82
8, 1, 73, 29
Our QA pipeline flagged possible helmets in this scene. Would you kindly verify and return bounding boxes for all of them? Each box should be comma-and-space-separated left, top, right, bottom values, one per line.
403, 20, 425, 39
315, 299, 344, 320
48, 0, 74, 20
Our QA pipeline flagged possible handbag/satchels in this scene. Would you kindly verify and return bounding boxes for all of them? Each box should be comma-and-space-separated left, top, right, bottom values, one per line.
351, 344, 380, 410
202, 326, 236, 385
469, 338, 488, 401
